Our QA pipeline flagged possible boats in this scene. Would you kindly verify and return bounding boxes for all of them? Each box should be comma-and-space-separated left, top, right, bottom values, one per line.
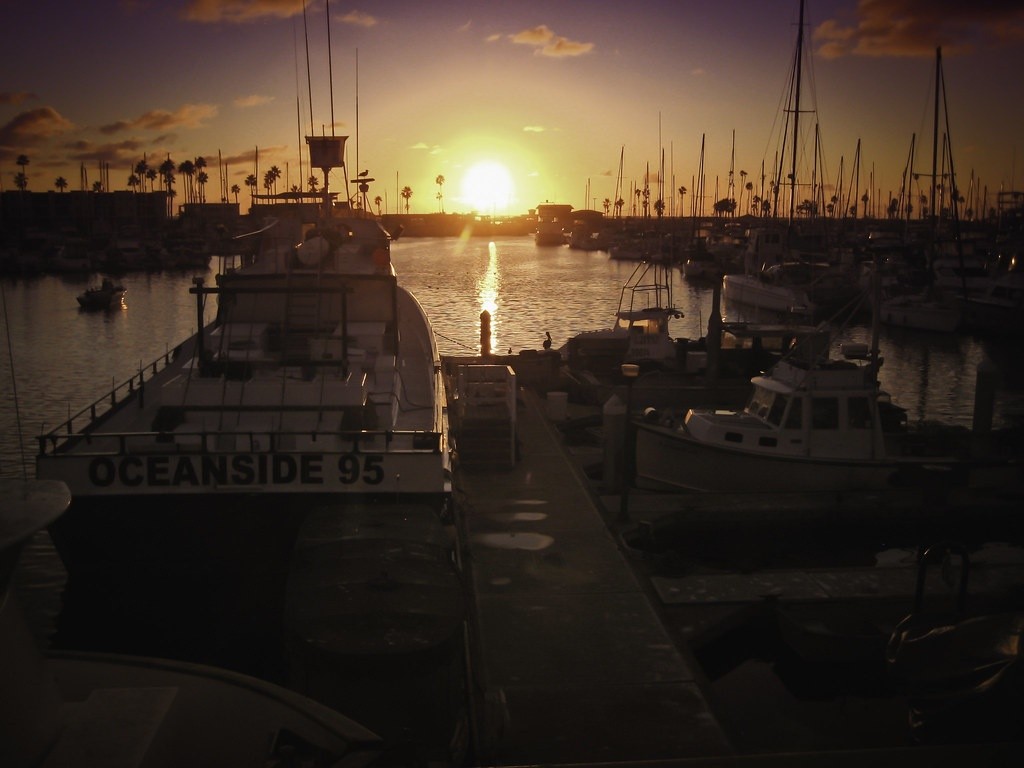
3, 0, 1023, 748
32, 0, 478, 664
